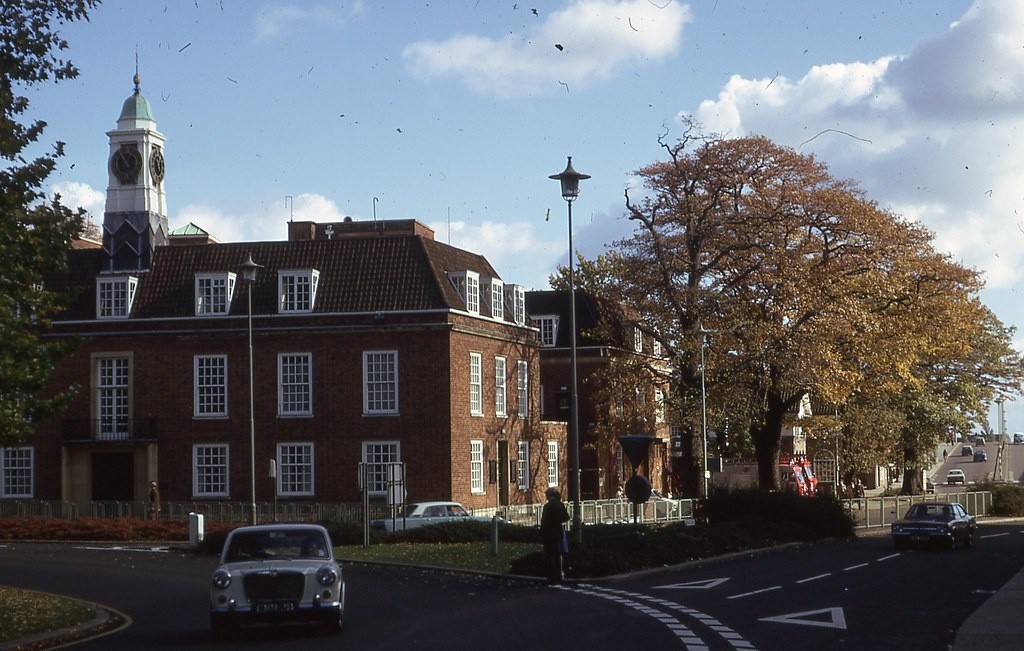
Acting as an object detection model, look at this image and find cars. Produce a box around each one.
[372,501,505,536]
[962,445,973,456]
[1014,432,1024,445]
[927,477,936,494]
[888,502,976,551]
[973,450,988,462]
[975,437,985,448]
[208,521,346,639]
[946,468,966,486]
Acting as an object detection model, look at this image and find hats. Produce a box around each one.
[544,488,561,499]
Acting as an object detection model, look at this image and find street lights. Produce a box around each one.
[694,319,718,495]
[548,154,591,555]
[237,248,265,525]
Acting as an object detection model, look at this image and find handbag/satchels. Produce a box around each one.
[559,524,570,559]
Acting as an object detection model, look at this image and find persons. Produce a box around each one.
[305,536,324,557]
[538,488,570,590]
[616,486,624,498]
[149,482,161,512]
[837,479,865,513]
[943,449,948,462]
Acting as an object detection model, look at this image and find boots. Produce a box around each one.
[544,562,561,583]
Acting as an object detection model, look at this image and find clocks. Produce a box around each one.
[111,146,142,180]
[148,149,165,184]
